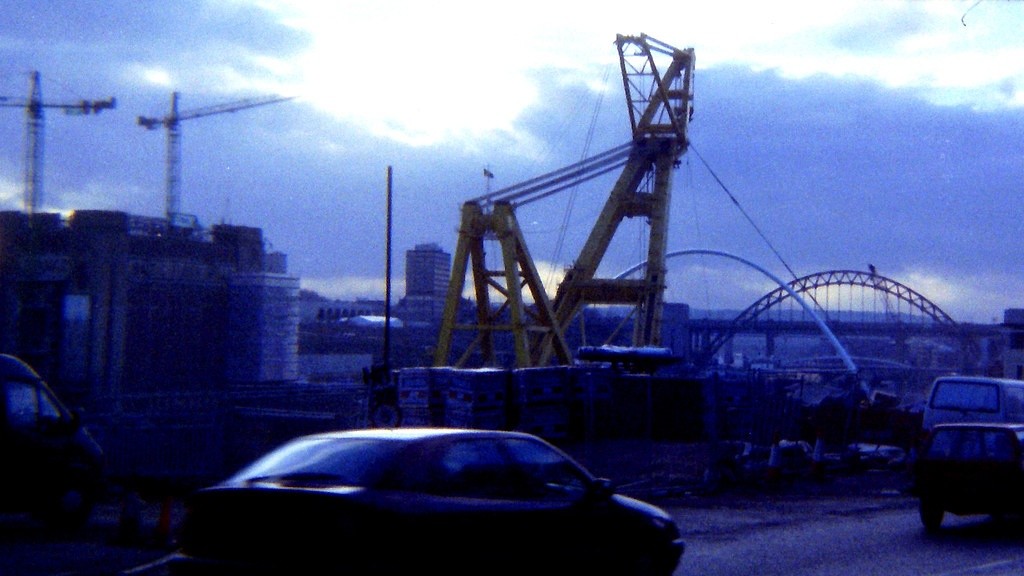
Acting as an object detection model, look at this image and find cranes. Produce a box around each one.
[1,72,114,215]
[138,94,290,225]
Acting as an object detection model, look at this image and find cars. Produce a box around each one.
[190,426,687,576]
[914,422,1023,534]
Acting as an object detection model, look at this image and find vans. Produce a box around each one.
[922,376,1023,431]
[0,352,107,539]
